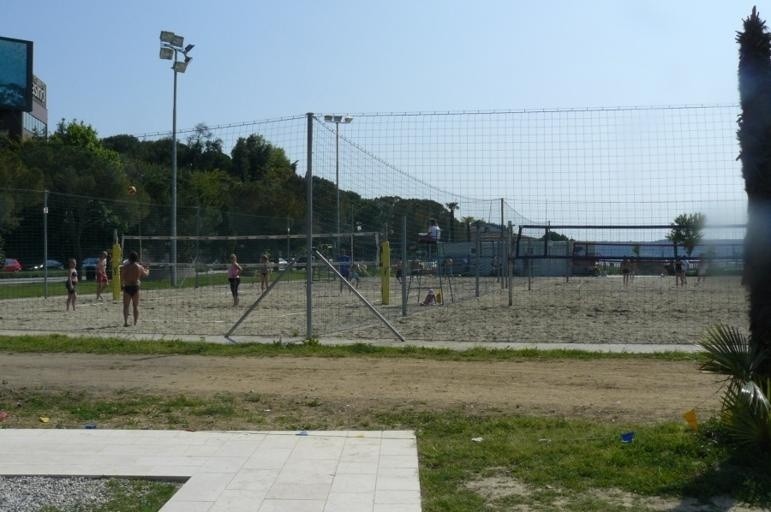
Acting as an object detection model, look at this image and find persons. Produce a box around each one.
[348,264,361,294]
[674,255,683,287]
[416,217,442,244]
[96,250,109,300]
[259,255,272,294]
[228,253,244,307]
[121,251,149,327]
[678,254,690,288]
[65,258,79,311]
[336,247,353,296]
[620,255,633,286]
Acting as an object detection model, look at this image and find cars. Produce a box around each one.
[0,256,129,273]
[263,258,288,269]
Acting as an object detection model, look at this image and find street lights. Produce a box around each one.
[160,29,194,289]
[325,115,353,263]
